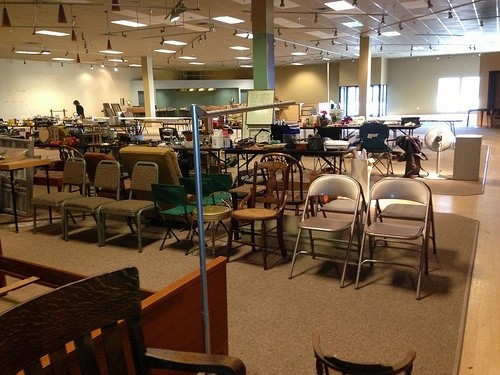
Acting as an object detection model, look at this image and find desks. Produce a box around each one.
[222,142,356,197]
[300,122,421,150]
[467,108,496,128]
[0,158,52,233]
[455,134,484,181]
[144,141,221,178]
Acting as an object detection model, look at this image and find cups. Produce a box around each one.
[212,136,224,149]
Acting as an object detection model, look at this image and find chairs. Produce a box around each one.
[0,117,441,375]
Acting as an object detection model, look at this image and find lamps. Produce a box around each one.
[334,29,338,36]
[169,12,180,22]
[107,39,112,49]
[280,0,285,7]
[279,38,355,63]
[57,0,67,23]
[381,17,386,25]
[313,14,318,23]
[1,3,12,27]
[167,34,207,73]
[71,27,77,41]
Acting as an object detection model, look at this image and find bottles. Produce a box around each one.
[278,119,285,126]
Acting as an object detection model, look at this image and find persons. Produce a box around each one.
[73,100,85,119]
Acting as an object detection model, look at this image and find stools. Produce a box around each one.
[193,206,231,254]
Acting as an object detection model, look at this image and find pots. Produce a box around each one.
[308,138,325,151]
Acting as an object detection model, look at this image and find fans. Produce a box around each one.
[424,127,454,180]
